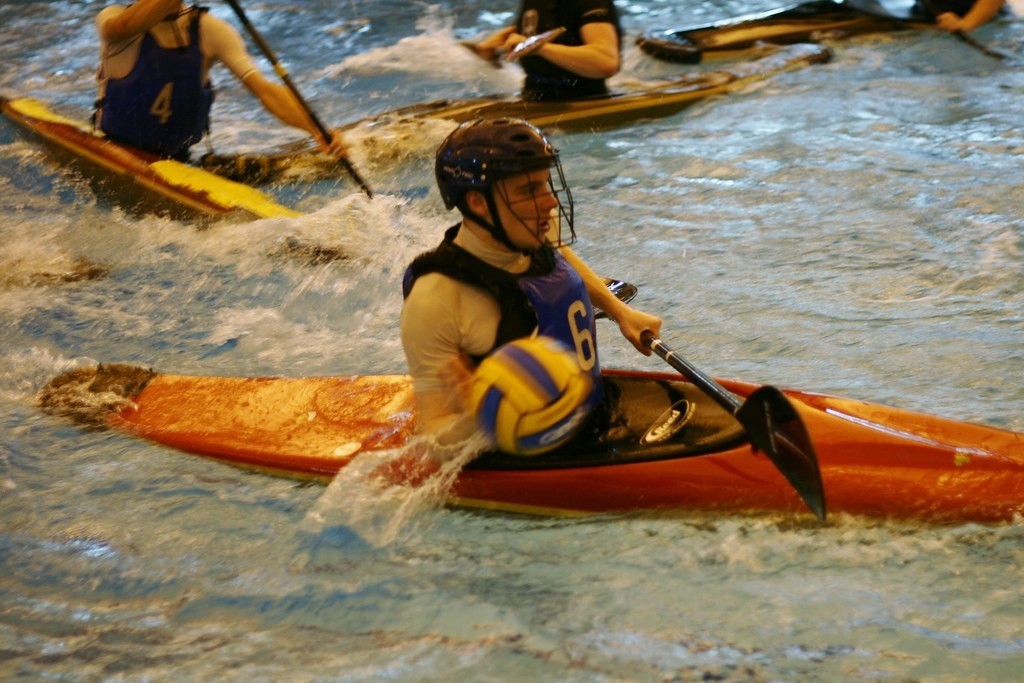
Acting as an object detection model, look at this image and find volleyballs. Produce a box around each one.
[469,336,587,458]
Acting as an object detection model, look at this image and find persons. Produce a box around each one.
[89,0,349,181]
[399,115,662,464]
[909,0,1008,31]
[473,0,624,102]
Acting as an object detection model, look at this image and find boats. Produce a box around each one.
[197,41,832,193]
[633,0,922,68]
[0,86,306,226]
[37,360,1024,525]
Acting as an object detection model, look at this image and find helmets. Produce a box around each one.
[435,116,562,210]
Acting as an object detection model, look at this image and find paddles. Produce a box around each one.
[225,0,376,201]
[915,1,1011,61]
[457,26,568,70]
[589,275,832,522]
